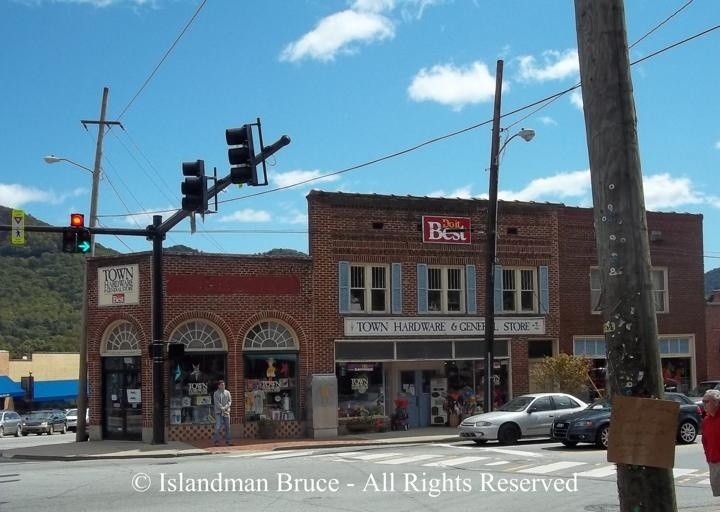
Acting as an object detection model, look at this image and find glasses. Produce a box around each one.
[702,399,709,403]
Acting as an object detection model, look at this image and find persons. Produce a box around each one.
[213,380,234,446]
[702,389,720,497]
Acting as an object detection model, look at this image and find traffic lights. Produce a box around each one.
[181,158,209,214]
[225,123,259,185]
[62,213,92,254]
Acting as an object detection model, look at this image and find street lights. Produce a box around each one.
[42,154,101,443]
[482,128,538,418]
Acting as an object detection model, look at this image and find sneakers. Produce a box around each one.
[215,441,232,446]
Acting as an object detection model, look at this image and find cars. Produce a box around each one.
[0,405,92,437]
[458,392,604,447]
[550,392,707,450]
